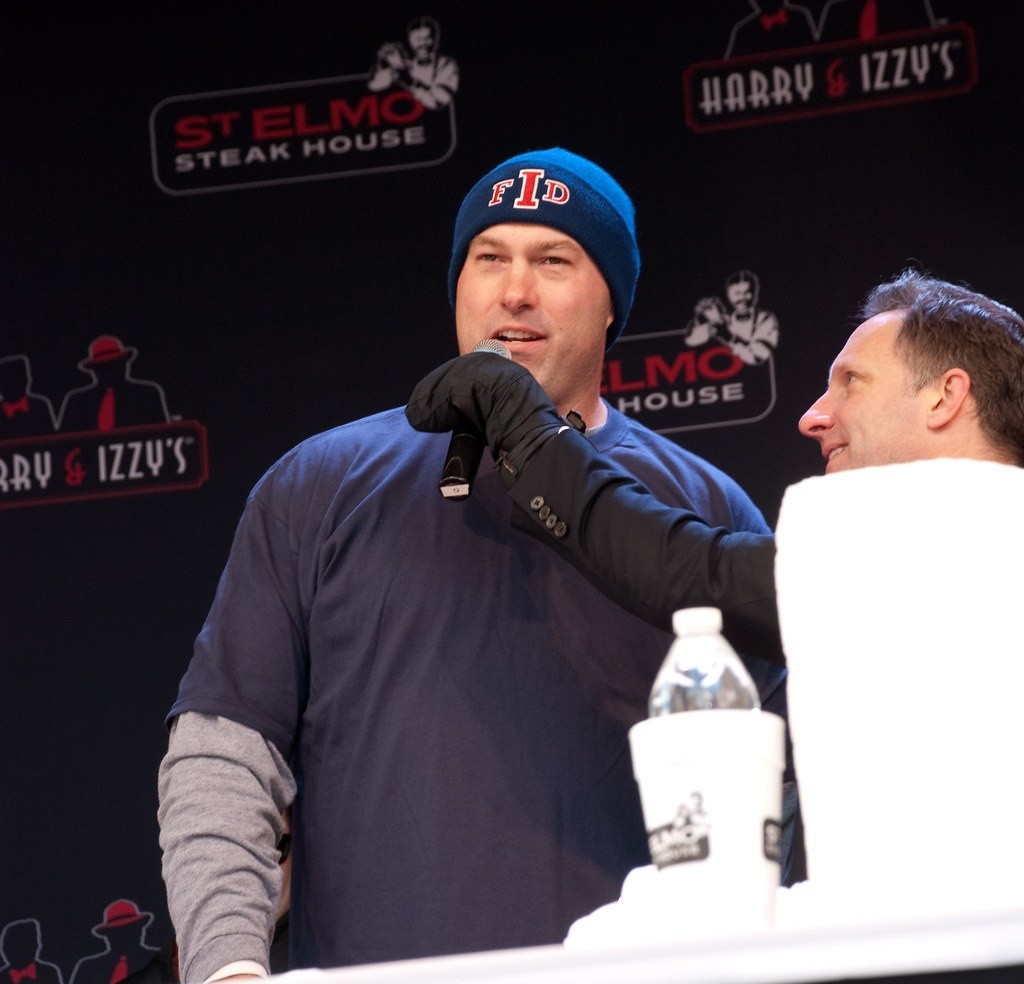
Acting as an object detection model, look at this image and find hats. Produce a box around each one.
[446,147,640,353]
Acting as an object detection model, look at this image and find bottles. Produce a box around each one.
[649,606,760,717]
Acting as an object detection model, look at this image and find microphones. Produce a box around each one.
[439,339,512,503]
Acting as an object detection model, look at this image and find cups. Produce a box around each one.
[627,710,787,892]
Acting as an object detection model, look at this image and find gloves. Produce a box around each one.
[406,351,567,486]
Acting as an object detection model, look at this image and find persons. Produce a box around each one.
[406,265,1023,667]
[157,144,802,984]
[116,807,292,984]
